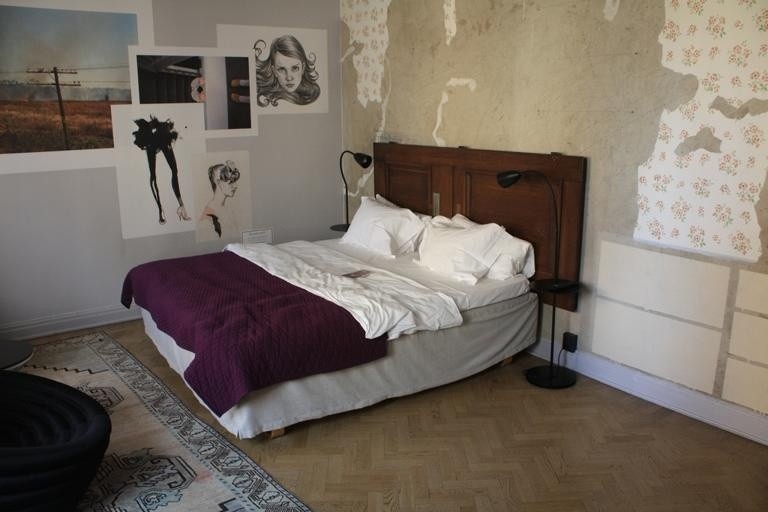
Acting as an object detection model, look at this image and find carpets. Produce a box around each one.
[17,327,312,511]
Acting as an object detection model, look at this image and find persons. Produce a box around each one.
[253,33,321,107]
[199,160,241,219]
[133,115,193,225]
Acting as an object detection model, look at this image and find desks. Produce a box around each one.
[0,338,34,372]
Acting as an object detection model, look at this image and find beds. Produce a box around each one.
[120,141,588,441]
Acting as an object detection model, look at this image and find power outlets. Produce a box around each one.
[562,332,578,353]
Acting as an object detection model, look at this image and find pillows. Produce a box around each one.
[454,213,533,282]
[416,217,501,284]
[342,197,425,259]
[376,194,429,254]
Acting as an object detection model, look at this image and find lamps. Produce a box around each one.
[340,151,372,226]
[497,170,582,390]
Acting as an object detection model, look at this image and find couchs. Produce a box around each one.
[1,372,112,511]
